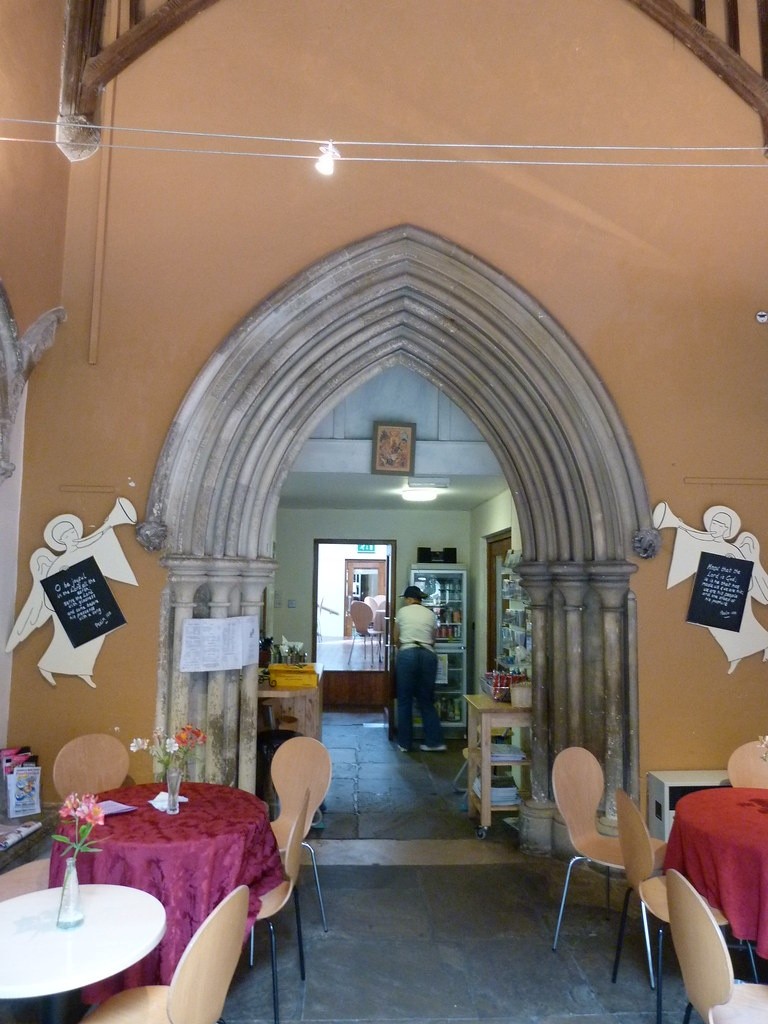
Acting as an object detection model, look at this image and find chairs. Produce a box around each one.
[609,787,759,1024]
[246,736,333,965]
[258,784,314,1024]
[76,884,251,1024]
[347,594,386,668]
[727,739,768,789]
[53,734,129,807]
[550,745,670,990]
[665,867,768,1024]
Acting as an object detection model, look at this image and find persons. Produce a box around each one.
[393,586,447,752]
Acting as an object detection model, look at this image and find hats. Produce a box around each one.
[398,586,421,598]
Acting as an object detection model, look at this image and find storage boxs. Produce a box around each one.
[416,547,457,564]
[479,676,510,701]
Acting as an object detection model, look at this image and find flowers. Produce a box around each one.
[48,792,116,858]
[129,720,209,782]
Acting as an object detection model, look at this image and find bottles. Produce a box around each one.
[415,577,462,600]
[441,697,461,722]
[259,631,307,665]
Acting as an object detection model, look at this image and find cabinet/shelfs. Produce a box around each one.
[408,563,468,728]
[495,553,532,668]
[462,692,531,840]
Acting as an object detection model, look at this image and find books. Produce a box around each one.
[0,746,41,818]
[472,742,526,806]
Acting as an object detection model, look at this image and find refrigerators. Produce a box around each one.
[408,563,473,740]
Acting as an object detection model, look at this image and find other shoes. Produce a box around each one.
[420,745,446,751]
[398,745,409,752]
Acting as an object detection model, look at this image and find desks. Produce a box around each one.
[258,684,314,731]
[374,609,386,631]
[662,787,768,983]
[46,779,284,1007]
[0,883,168,1024]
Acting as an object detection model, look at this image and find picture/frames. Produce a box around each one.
[371,419,417,476]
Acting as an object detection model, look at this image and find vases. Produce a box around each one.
[165,769,183,816]
[57,857,86,929]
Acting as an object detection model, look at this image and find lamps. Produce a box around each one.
[314,138,340,177]
[399,477,450,502]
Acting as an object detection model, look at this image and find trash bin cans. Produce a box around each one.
[257,730,305,776]
